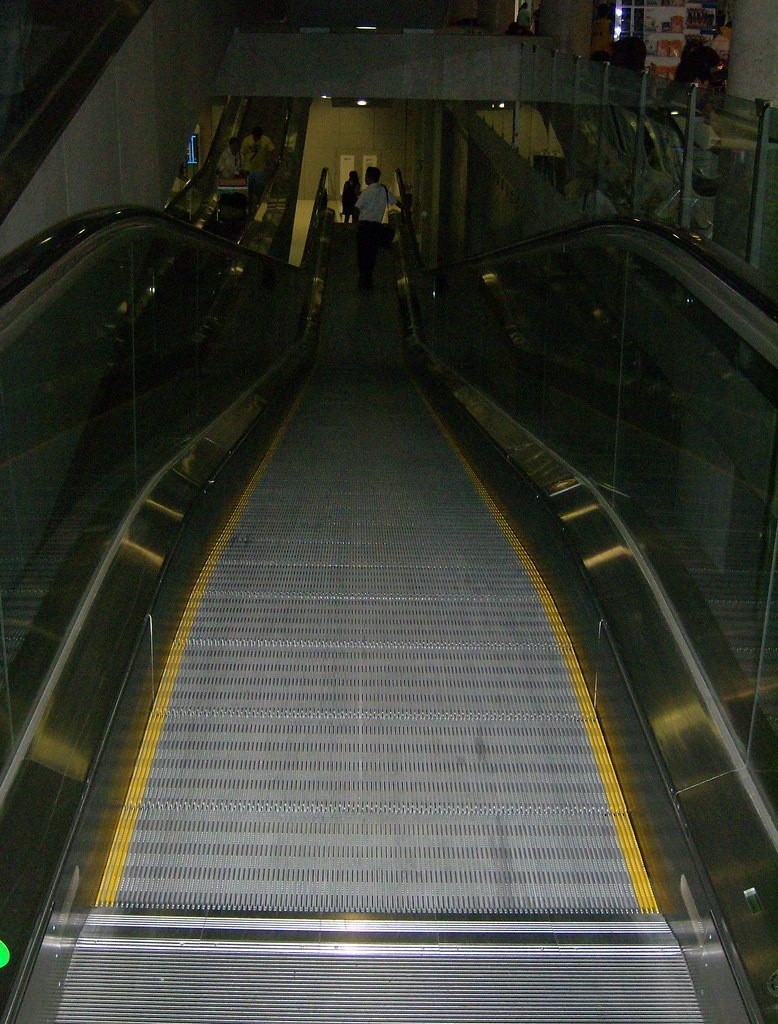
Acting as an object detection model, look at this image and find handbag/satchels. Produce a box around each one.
[379,223,393,250]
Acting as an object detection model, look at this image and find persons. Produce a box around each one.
[341,171,362,224]
[354,166,412,288]
[217,136,250,178]
[239,125,275,175]
[517,2,617,53]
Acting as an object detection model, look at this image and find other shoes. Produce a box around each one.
[357,281,374,293]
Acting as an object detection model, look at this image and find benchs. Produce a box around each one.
[549,75,721,236]
